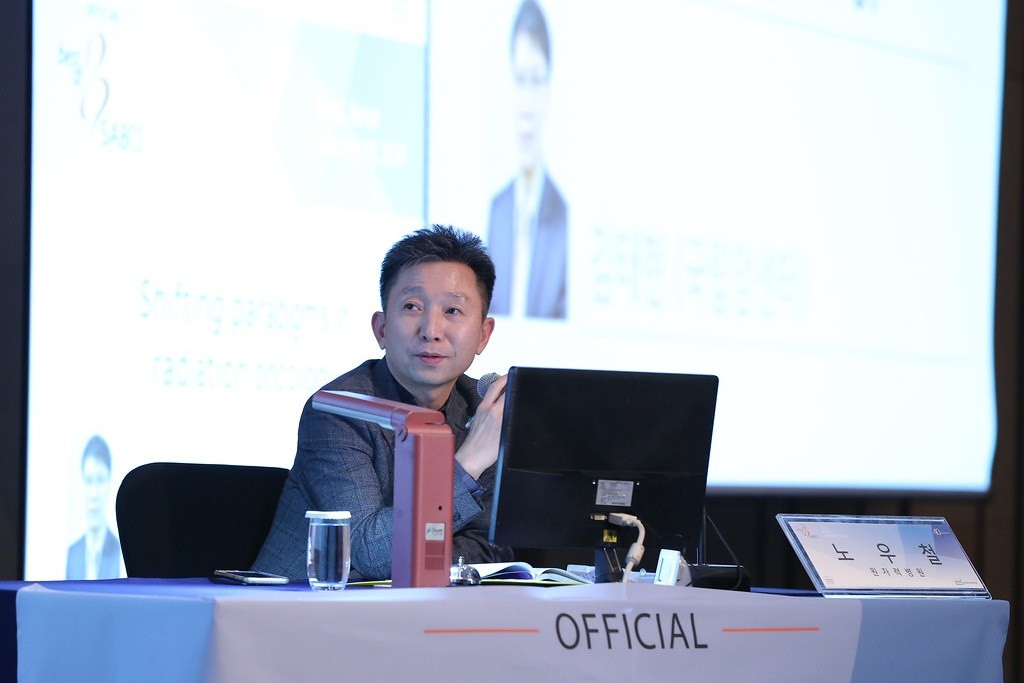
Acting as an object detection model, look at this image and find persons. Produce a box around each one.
[482,0,569,321]
[248,224,508,587]
[64,434,120,581]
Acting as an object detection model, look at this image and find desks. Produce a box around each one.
[0,579,1010,683]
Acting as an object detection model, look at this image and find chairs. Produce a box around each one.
[116,463,291,580]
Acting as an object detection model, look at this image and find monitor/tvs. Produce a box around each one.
[488,367,719,584]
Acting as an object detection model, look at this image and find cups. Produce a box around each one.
[307,510,351,592]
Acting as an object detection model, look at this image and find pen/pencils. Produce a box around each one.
[464,383,508,428]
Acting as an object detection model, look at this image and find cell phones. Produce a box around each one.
[214,568,289,584]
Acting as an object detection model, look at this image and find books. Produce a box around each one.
[450,559,599,594]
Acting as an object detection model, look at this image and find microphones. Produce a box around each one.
[477,371,507,401]
